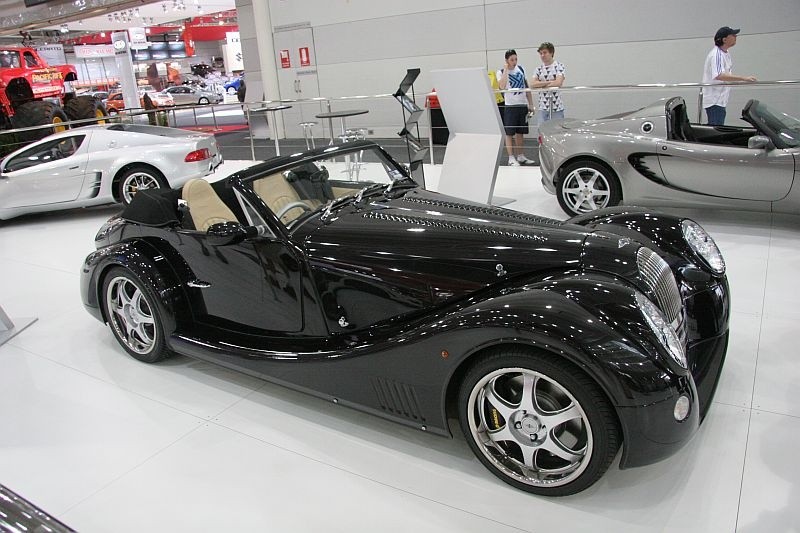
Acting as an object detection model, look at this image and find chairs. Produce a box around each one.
[255,174,304,224]
[182,179,240,232]
[680,112,696,142]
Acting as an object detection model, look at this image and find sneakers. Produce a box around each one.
[508,159,520,166]
[517,156,535,164]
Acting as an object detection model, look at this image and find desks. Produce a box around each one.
[250,105,292,158]
[317,110,368,164]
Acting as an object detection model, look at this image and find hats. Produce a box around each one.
[714,27,740,41]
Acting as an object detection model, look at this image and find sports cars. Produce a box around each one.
[0,122,224,222]
[537,96,799,218]
[80,137,732,498]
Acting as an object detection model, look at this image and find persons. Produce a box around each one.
[496,49,536,166]
[702,27,757,126]
[237,80,248,126]
[529,42,566,124]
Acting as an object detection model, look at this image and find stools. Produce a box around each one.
[299,121,374,182]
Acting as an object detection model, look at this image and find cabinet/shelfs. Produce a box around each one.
[392,68,430,190]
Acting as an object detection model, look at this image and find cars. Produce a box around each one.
[75,55,246,109]
[0,47,79,123]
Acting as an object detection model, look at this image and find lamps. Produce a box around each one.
[107,7,154,26]
[78,20,84,26]
[162,0,202,14]
[60,22,68,33]
